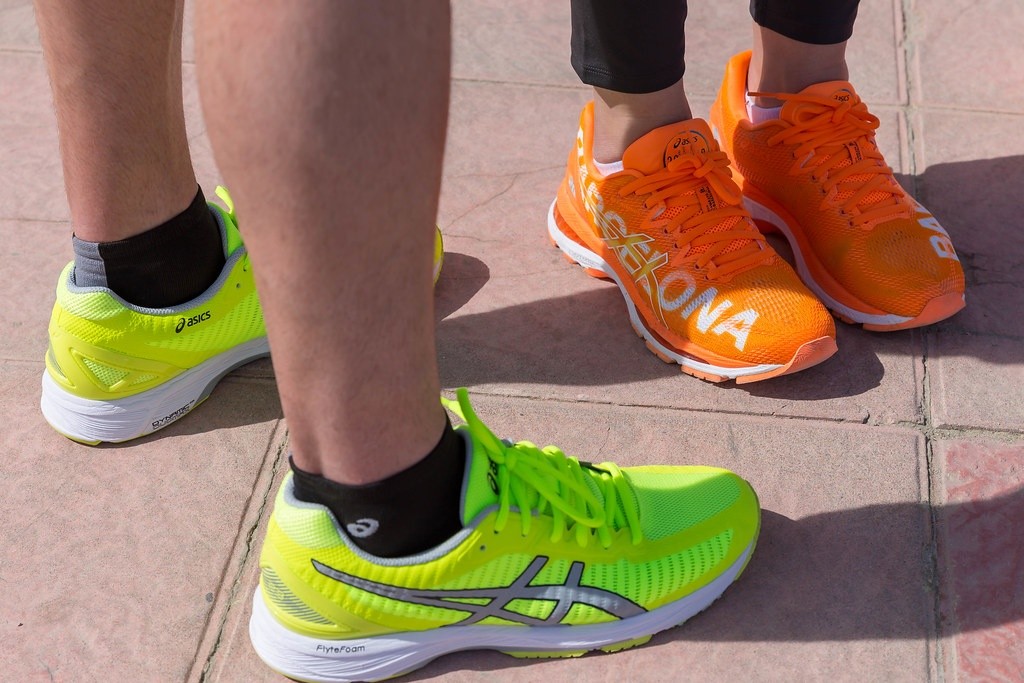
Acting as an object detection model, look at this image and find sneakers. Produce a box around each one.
[545,102,840,385]
[248,389,761,683]
[709,49,967,332]
[41,185,443,448]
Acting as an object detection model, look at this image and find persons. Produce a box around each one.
[41,1,761,681]
[547,0,967,384]
